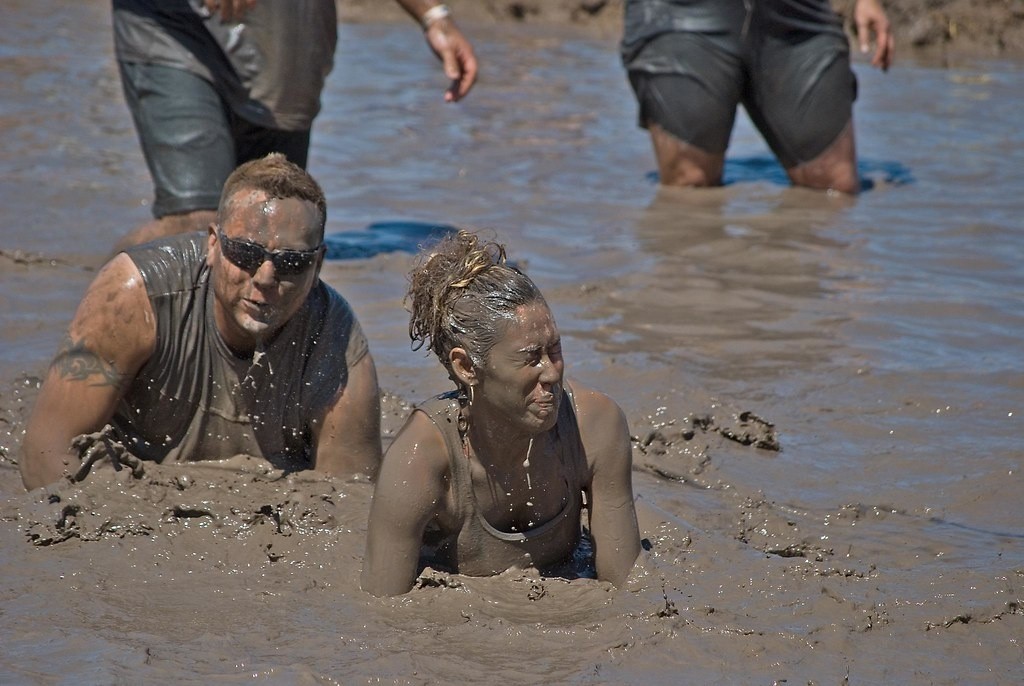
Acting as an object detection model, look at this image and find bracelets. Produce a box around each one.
[421,4,450,29]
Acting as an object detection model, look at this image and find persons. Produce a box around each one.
[111,0,478,255]
[620,0,895,196]
[20,153,382,493]
[359,229,642,597]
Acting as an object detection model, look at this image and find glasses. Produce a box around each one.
[215,223,319,275]
